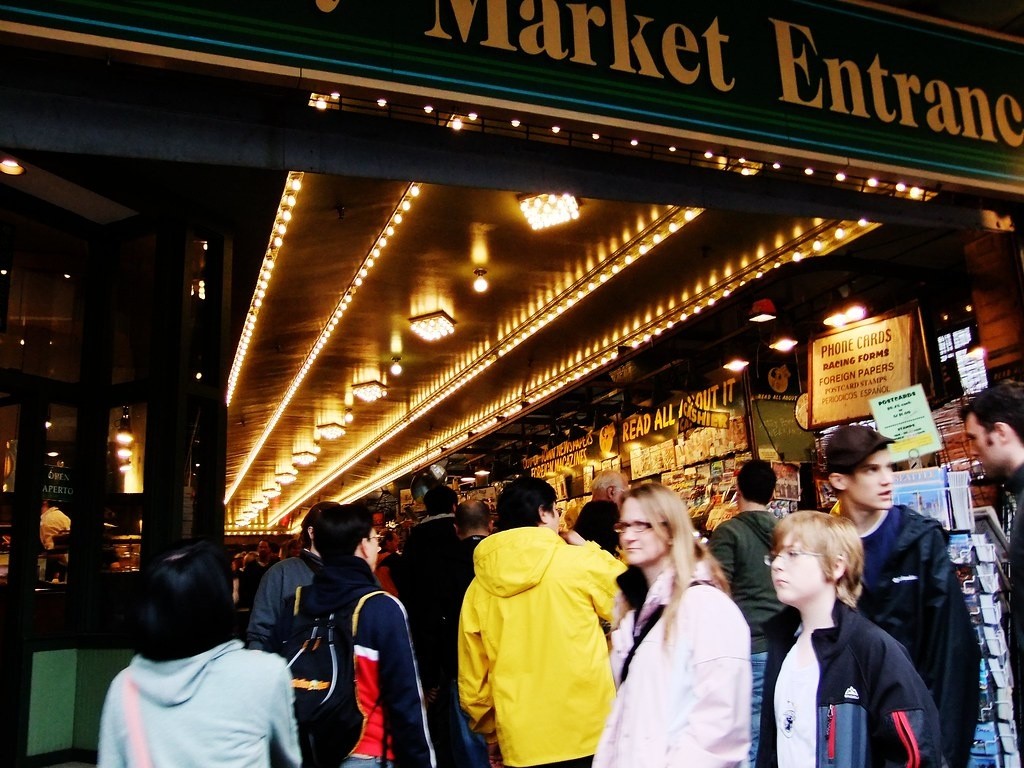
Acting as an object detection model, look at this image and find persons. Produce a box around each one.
[957,380,1024,768]
[227,425,983,768]
[39,494,72,585]
[98,543,303,768]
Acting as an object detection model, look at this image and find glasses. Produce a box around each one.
[544,505,562,517]
[615,521,669,533]
[763,553,825,564]
[364,533,384,542]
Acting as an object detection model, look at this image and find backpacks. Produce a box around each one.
[277,612,365,768]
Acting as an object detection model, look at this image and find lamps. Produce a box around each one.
[442,354,715,492]
[748,298,777,323]
[115,405,133,459]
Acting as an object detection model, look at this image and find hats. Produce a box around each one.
[825,425,896,475]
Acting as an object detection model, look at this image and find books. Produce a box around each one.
[542,417,1022,768]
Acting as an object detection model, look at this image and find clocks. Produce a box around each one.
[794,392,808,431]
